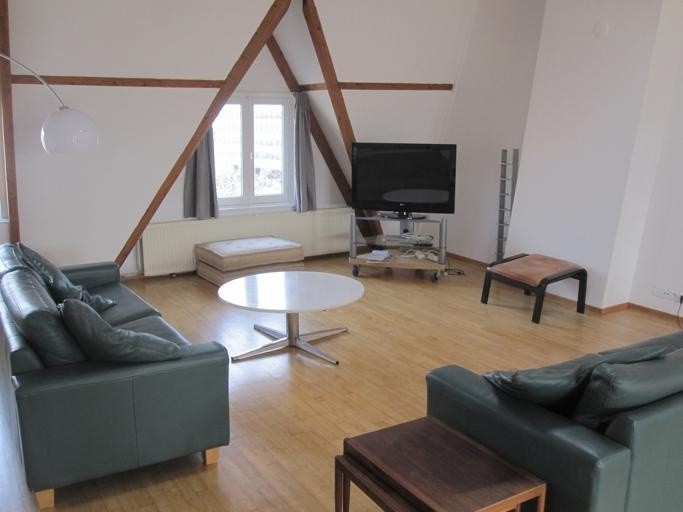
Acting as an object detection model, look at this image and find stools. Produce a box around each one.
[192,234,306,288]
[479,252,587,323]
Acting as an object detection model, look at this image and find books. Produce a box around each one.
[366,250,389,261]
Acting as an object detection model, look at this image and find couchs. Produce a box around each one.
[0,239,231,511]
[426,321,682,512]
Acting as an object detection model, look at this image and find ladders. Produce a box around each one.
[496,148,518,262]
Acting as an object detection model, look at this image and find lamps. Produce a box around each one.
[0,48,100,161]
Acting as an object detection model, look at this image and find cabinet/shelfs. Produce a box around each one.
[347,210,450,284]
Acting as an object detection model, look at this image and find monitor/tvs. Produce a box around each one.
[351,142,457,219]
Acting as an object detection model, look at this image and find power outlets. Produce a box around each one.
[661,287,683,304]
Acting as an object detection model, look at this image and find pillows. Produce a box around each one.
[14,242,117,311]
[59,298,181,367]
[484,343,667,406]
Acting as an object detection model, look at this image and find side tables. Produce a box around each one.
[334,412,549,510]
